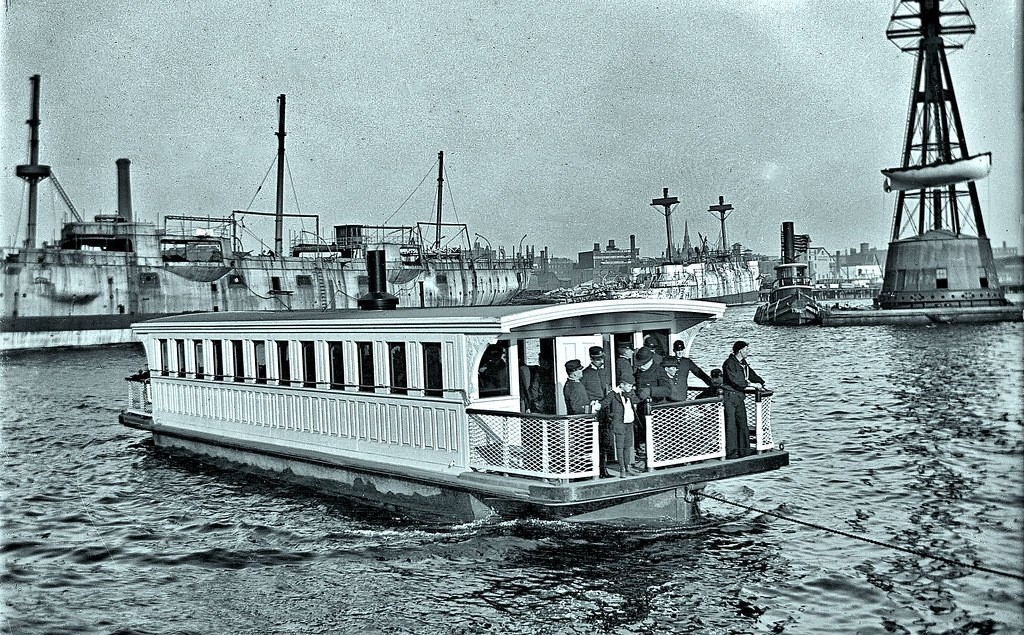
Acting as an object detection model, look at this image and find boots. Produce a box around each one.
[599,453,615,479]
[617,448,640,478]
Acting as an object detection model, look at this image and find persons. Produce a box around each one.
[477,333,774,478]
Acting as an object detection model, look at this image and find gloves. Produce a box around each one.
[590,400,601,410]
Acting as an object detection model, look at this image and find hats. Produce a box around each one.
[538,352,551,361]
[732,341,748,350]
[644,337,659,347]
[619,342,635,350]
[673,340,685,350]
[589,346,606,357]
[710,369,722,378]
[620,373,636,384]
[660,356,681,366]
[635,347,654,366]
[565,359,584,371]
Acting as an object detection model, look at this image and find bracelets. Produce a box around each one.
[763,385,765,386]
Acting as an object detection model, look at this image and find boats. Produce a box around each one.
[117,250,789,530]
[511,271,627,305]
[605,188,766,306]
[754,221,826,325]
[0,74,535,352]
